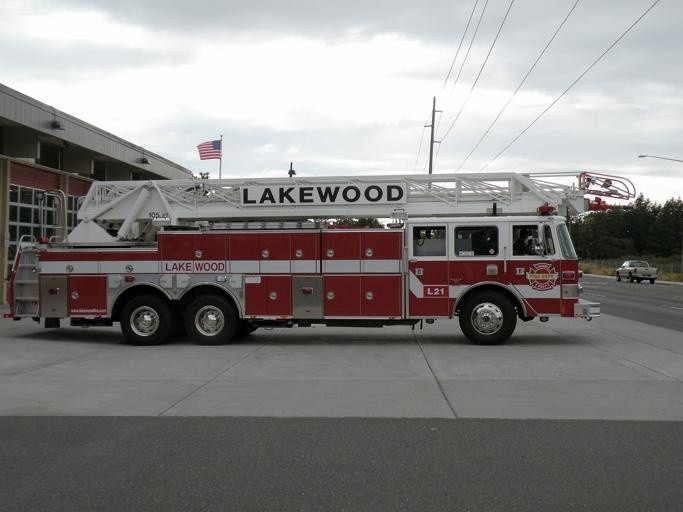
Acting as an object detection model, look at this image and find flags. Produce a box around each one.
[197,139,221,161]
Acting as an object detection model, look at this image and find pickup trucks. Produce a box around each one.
[614,258,660,286]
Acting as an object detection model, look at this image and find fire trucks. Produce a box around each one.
[5,170,642,347]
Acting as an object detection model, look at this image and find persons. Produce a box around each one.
[513,229,537,255]
